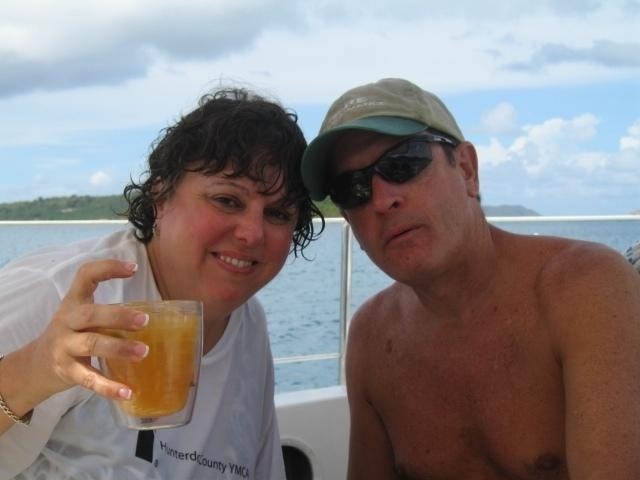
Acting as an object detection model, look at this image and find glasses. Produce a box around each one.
[328,136,457,210]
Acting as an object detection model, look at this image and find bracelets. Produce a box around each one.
[0,354,33,427]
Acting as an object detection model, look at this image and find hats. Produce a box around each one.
[300,79,466,201]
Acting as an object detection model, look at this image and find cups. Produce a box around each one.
[96,299,208,432]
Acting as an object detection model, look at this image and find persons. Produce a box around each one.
[300,77,640,480]
[0,86,325,480]
